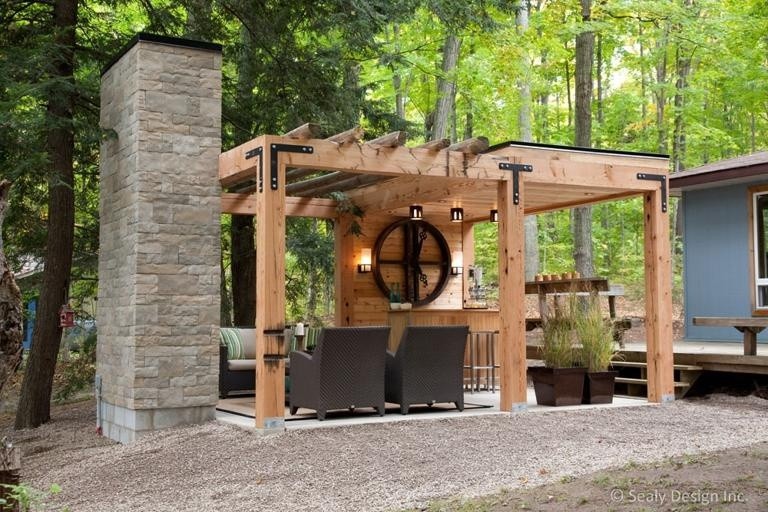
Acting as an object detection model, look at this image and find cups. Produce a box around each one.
[295,323,304,335]
[535,273,581,282]
[390,302,412,310]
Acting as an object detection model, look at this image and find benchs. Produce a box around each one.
[524,277,632,347]
[692,316,768,356]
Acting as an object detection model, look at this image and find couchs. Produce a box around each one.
[219,323,314,399]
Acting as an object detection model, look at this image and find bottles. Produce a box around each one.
[390,282,401,303]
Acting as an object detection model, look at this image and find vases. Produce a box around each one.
[533,272,580,284]
[400,301,412,311]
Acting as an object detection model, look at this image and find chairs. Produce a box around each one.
[289,324,470,422]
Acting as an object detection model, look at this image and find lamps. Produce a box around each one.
[451,249,464,277]
[357,247,372,274]
[408,205,499,223]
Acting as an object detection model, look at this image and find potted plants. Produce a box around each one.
[388,281,402,310]
[526,278,624,407]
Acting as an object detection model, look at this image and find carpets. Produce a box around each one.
[214,399,495,422]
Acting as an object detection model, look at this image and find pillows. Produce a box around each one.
[220,327,246,360]
